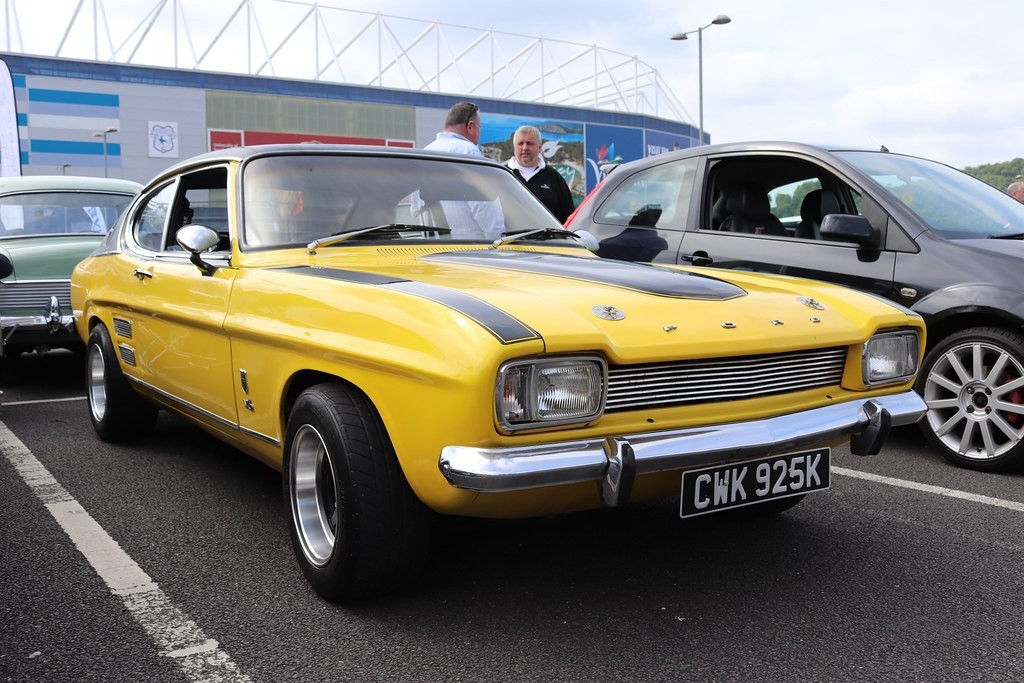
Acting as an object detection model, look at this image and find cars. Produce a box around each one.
[0,175,193,363]
[558,143,1023,474]
[69,144,927,606]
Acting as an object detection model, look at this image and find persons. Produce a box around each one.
[597,204,668,263]
[1007,182,1024,203]
[410,102,506,240]
[504,126,575,225]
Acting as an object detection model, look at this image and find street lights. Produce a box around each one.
[670,14,731,146]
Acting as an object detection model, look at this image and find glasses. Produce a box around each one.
[466,102,478,128]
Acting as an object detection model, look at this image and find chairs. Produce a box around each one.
[719,185,789,238]
[340,195,402,232]
[796,189,842,241]
[23,216,72,231]
